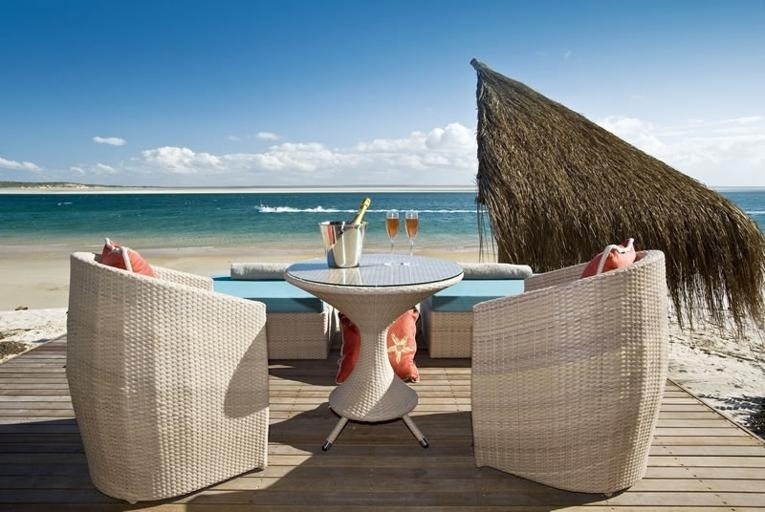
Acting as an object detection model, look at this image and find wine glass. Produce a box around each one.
[383,211,419,267]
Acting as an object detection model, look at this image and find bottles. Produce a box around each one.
[351,197,371,225]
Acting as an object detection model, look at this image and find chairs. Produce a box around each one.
[64,253,271,506]
[470,248,673,500]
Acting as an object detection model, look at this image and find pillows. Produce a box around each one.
[101,236,157,277]
[578,237,638,276]
[334,302,421,385]
[459,262,534,280]
[230,261,288,281]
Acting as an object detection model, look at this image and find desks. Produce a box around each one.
[282,255,466,453]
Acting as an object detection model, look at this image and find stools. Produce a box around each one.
[207,274,334,362]
[420,278,524,358]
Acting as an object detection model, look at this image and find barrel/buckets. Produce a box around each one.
[320,220,367,270]
